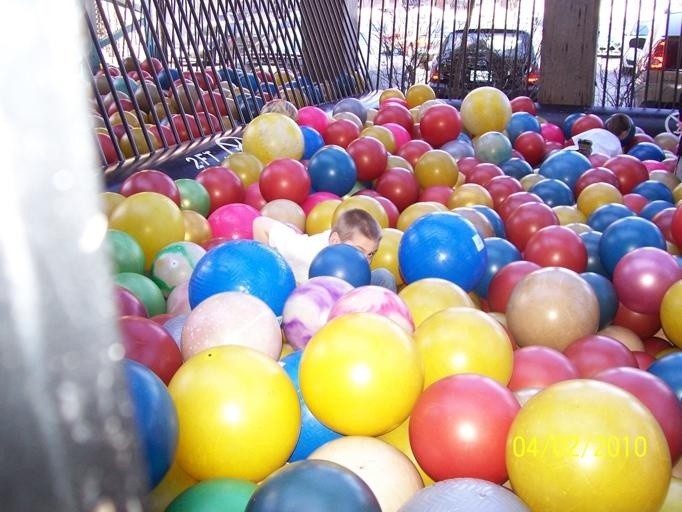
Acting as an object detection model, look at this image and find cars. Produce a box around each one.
[623,37,653,73]
[167,23,241,65]
[241,29,304,66]
[601,46,619,56]
[372,6,459,55]
[633,36,682,107]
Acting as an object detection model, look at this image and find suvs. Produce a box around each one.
[429,30,538,101]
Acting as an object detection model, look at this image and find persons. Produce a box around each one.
[544,112,634,167]
[251,208,383,280]
